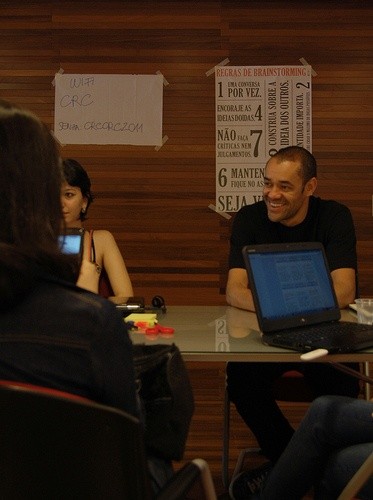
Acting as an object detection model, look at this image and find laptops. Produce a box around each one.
[243,242,373,355]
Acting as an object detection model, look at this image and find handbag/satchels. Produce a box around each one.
[131,343,195,463]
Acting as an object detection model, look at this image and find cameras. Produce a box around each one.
[54,228,83,260]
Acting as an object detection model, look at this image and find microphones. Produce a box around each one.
[152,296,167,312]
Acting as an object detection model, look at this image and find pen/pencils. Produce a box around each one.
[126,320,142,331]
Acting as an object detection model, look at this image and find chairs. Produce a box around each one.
[225,360,373,500]
[0,379,151,500]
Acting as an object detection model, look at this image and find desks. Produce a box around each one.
[122,304,373,494]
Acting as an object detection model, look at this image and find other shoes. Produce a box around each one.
[230,463,273,500]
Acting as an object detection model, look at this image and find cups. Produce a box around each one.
[356,299,373,325]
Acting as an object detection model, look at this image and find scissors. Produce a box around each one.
[145,321,174,335]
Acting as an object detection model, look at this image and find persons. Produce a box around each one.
[260,397,373,500]
[1,101,208,500]
[224,146,359,500]
[57,158,133,305]
